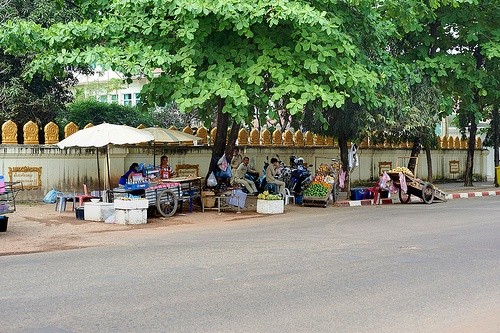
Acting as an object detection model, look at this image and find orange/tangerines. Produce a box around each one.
[311,181,331,188]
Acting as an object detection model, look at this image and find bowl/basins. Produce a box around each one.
[90,199,100,203]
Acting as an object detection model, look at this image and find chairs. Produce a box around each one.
[283,188,295,206]
[52,184,93,212]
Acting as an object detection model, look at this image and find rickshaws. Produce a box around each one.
[384,156,447,204]
[107,176,204,217]
[300,155,341,207]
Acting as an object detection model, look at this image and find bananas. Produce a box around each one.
[257,190,282,199]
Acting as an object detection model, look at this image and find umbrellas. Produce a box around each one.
[139,127,204,171]
[57,121,154,202]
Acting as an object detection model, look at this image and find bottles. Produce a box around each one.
[126,178,147,184]
[163,166,168,171]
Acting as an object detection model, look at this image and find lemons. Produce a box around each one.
[304,184,328,198]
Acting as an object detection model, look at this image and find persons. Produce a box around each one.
[118,163,139,188]
[230,146,260,196]
[155,155,173,179]
[264,154,304,196]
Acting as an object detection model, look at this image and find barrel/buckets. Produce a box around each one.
[0,215,9,231]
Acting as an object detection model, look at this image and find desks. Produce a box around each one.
[161,176,202,212]
[201,196,240,213]
[109,183,180,217]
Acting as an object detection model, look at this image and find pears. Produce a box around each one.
[390,167,413,175]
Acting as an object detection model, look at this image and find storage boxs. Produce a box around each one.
[256,198,284,214]
[351,188,370,200]
[225,190,248,208]
[84,197,148,224]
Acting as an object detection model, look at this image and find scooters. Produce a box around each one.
[241,154,313,195]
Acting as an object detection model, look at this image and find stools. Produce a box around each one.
[266,183,277,194]
